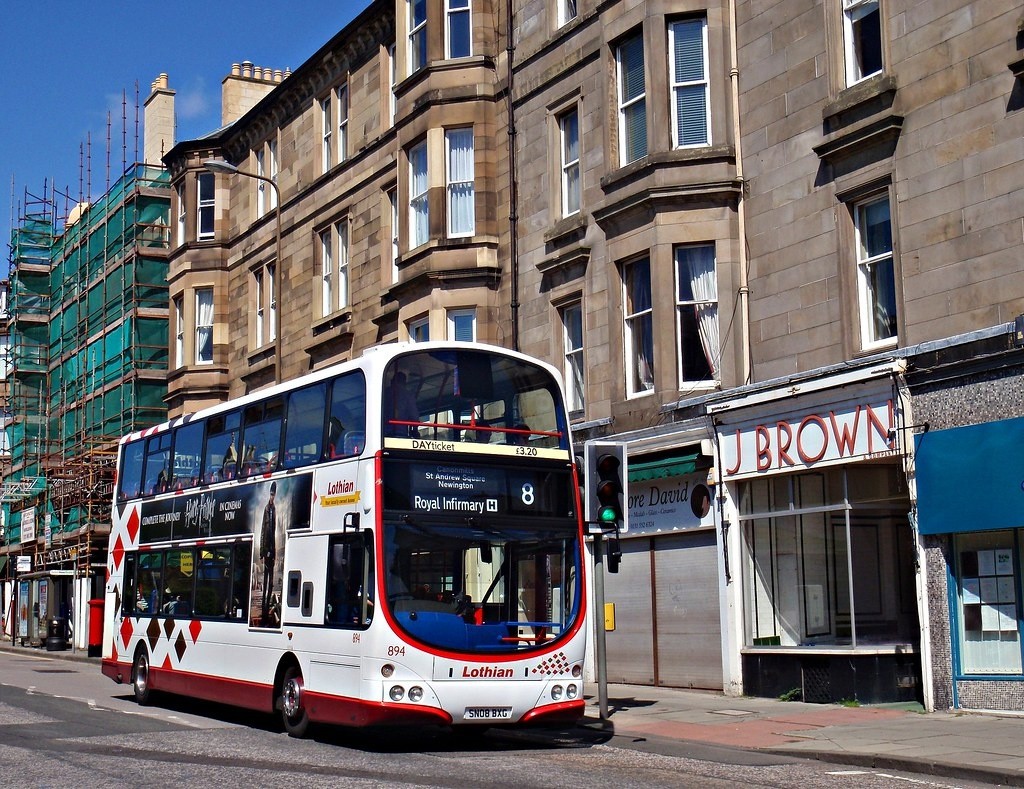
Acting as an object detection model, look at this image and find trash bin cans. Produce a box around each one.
[46,616,67,652]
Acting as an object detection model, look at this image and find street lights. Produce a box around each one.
[201,161,285,385]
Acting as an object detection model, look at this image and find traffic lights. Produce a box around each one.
[572,454,589,536]
[582,436,629,536]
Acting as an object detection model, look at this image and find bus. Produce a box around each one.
[98,340,625,741]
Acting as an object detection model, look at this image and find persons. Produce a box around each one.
[384,371,420,438]
[476,422,492,443]
[335,558,351,606]
[357,543,442,609]
[513,424,531,445]
[163,575,178,592]
[260,482,276,606]
